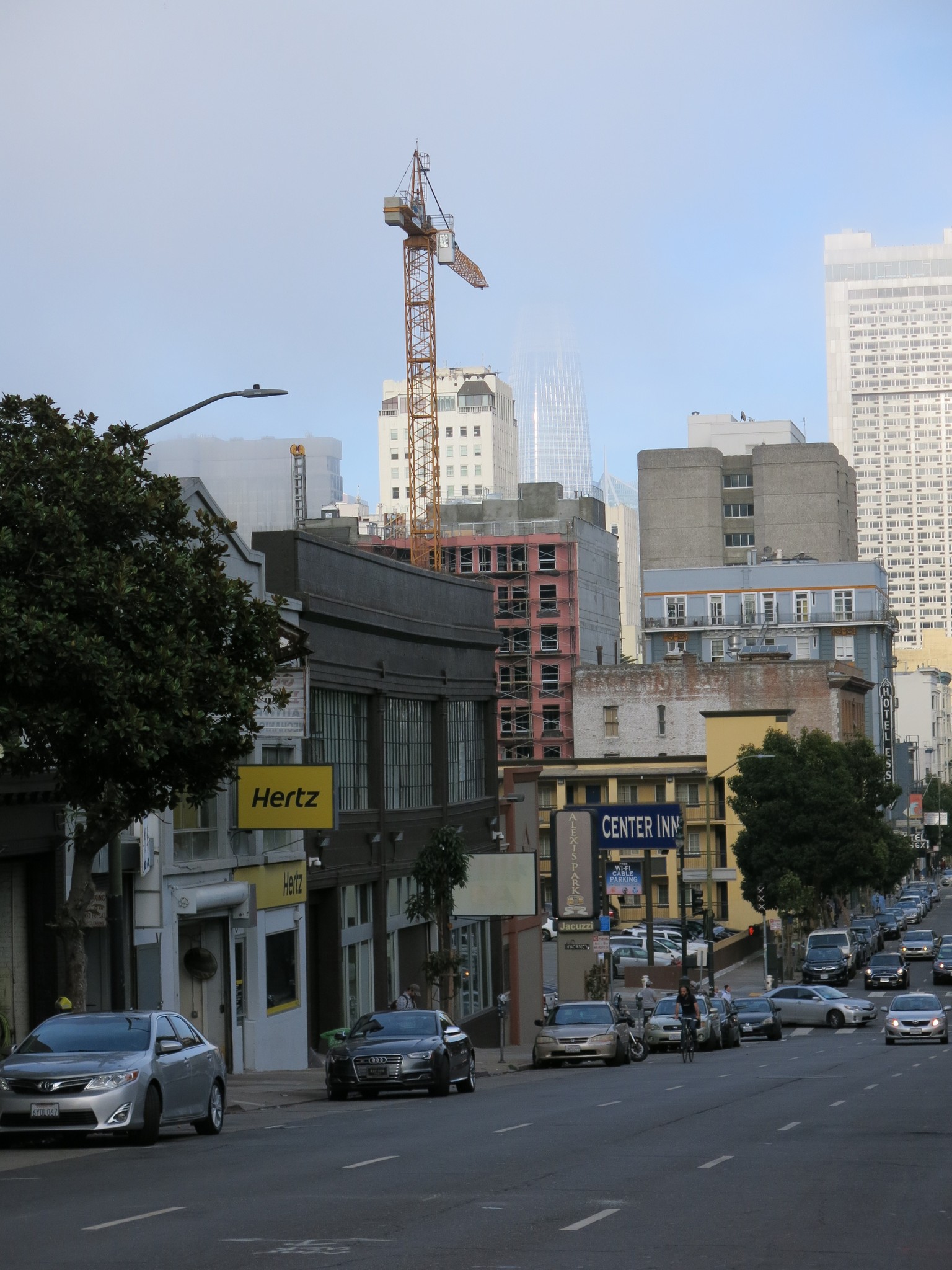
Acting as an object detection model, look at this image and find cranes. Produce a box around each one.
[382,139,489,572]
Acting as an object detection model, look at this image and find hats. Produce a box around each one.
[409,984,421,997]
[646,981,652,985]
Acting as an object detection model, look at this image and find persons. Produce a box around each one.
[395,984,421,1028]
[826,901,833,922]
[714,985,719,997]
[642,981,657,1027]
[673,985,700,1049]
[721,985,731,1004]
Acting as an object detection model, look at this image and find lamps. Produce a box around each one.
[229,829,253,875]
[262,833,324,854]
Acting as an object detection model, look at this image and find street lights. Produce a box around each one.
[705,754,777,997]
[97,384,286,1014]
[906,775,936,882]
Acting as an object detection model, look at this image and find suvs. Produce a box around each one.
[598,917,744,979]
[802,945,852,987]
[642,990,723,1052]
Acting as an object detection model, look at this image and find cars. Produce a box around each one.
[848,880,941,968]
[942,869,952,886]
[324,1008,477,1100]
[531,1000,631,1067]
[730,996,783,1040]
[600,900,621,929]
[863,951,911,990]
[755,983,879,1030]
[542,903,558,941]
[708,996,742,1050]
[932,933,952,985]
[882,993,952,1044]
[0,1009,226,1148]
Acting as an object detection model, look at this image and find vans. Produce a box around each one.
[805,927,861,970]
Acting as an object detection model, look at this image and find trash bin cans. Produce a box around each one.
[320,1027,351,1047]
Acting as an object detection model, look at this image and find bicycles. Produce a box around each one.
[672,1017,699,1063]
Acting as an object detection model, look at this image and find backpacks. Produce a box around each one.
[390,994,415,1011]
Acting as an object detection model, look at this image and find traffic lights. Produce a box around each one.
[691,888,704,915]
[748,925,762,938]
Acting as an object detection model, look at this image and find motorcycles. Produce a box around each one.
[614,993,648,1062]
